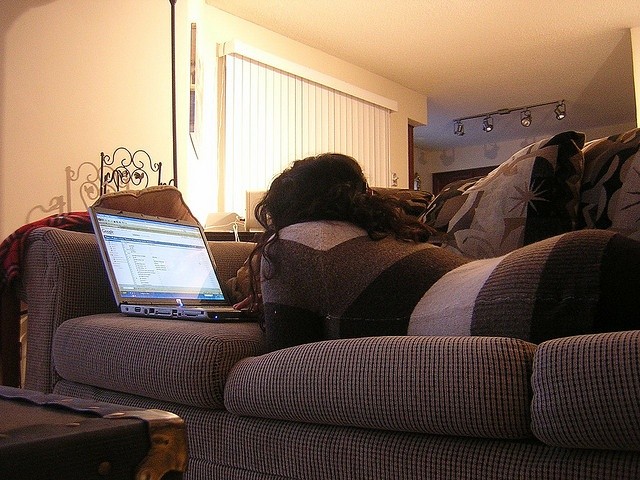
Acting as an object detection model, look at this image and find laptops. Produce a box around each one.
[88,207,254,322]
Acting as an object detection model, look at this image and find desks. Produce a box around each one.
[0,385,189,480]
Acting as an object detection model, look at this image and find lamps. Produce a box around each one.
[519,109,533,128]
[482,114,494,133]
[553,103,567,121]
[453,120,465,136]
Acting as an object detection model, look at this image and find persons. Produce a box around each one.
[232,152,639,353]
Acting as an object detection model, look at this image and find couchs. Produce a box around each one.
[20,220,640,479]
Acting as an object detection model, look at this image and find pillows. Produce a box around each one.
[415,128,586,259]
[365,185,433,232]
[580,127,640,238]
[90,187,202,228]
